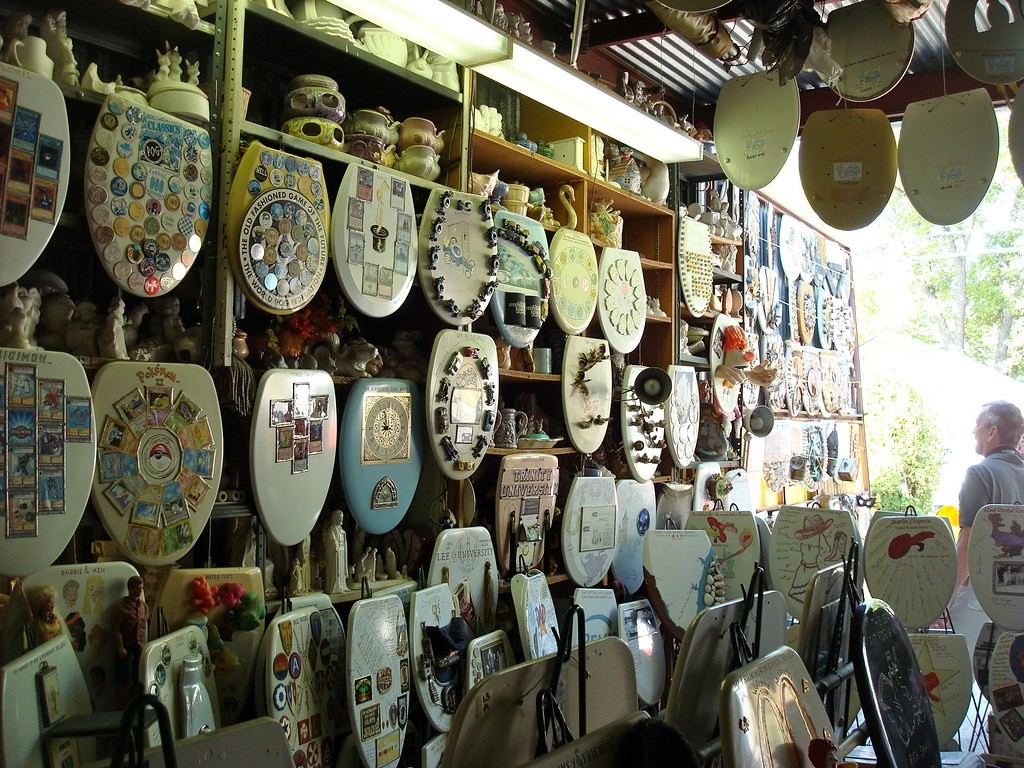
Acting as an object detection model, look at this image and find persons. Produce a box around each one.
[808,738,837,768]
[929,400,1024,628]
[0,9,713,710]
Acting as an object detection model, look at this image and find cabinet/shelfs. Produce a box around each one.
[0,0,868,768]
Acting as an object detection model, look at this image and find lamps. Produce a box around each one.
[329,0,704,164]
